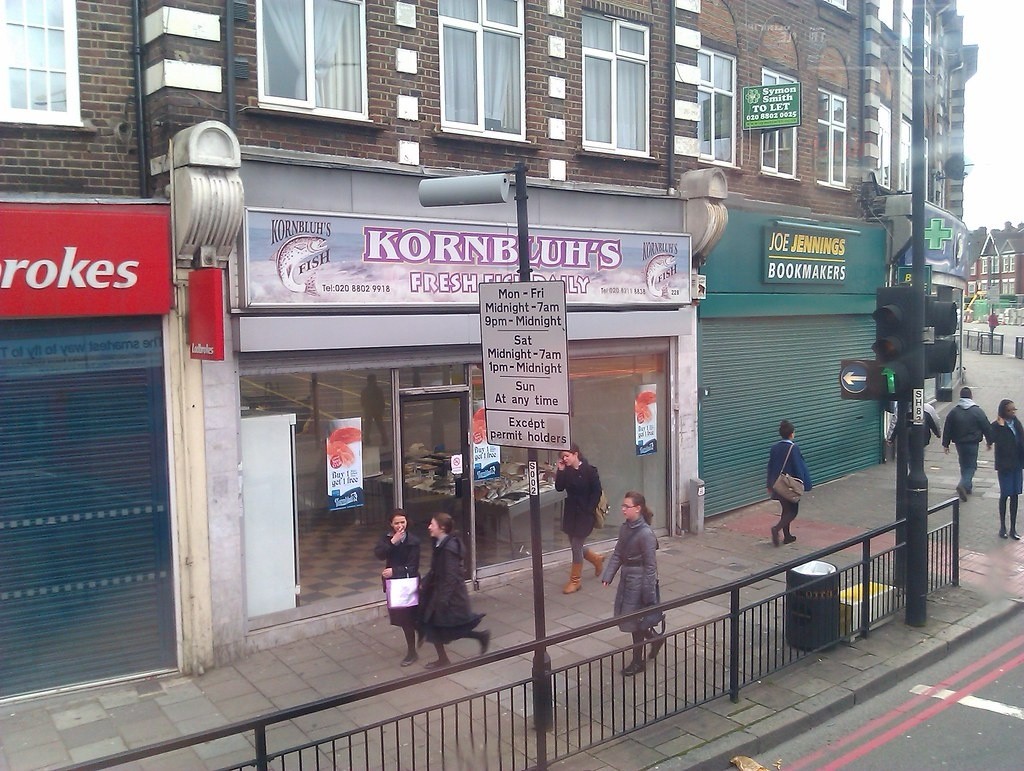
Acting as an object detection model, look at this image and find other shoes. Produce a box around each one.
[784,536,796,544]
[400,652,419,666]
[425,660,452,669]
[999,530,1008,538]
[622,660,647,676]
[772,526,780,545]
[479,630,490,653]
[966,490,973,494]
[1010,531,1020,540]
[957,486,968,502]
[649,634,664,658]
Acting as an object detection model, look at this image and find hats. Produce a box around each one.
[960,387,972,398]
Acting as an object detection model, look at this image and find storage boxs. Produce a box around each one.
[840,581,898,642]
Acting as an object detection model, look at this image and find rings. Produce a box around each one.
[399,528,401,530]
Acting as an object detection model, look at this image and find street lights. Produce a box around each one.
[418,174,555,728]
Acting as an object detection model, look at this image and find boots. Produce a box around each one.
[562,562,583,593]
[585,549,605,576]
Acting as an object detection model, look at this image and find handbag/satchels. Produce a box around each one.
[637,605,665,631]
[386,575,420,610]
[773,474,805,504]
[594,487,612,530]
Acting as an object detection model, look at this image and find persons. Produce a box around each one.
[361,373,388,445]
[416,513,489,669]
[943,387,992,501]
[766,420,812,545]
[987,399,1024,540]
[555,440,605,593]
[988,312,998,332]
[887,399,940,449]
[602,490,664,675]
[375,509,424,666]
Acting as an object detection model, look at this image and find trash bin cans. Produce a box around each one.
[1015,336,1024,359]
[786,559,841,651]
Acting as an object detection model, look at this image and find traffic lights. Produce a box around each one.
[841,287,924,401]
[925,297,958,379]
